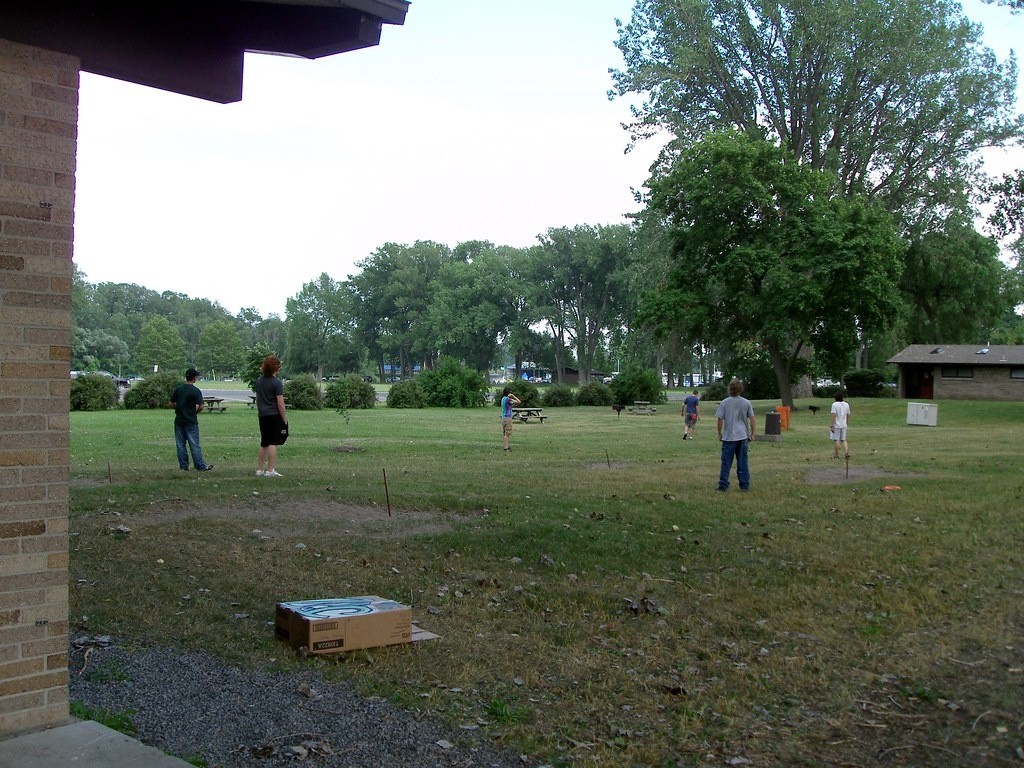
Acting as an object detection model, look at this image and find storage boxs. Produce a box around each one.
[273,596,440,657]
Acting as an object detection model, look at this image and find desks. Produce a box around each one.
[627,401,655,415]
[500,408,545,424]
[196,396,225,414]
[246,395,288,411]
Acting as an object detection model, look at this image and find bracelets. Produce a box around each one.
[830,425,834,427]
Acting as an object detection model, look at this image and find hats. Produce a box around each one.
[186,368,201,379]
[833,391,843,401]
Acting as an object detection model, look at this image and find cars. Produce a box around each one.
[811,378,847,390]
[126,375,144,381]
[70,370,131,391]
[488,375,542,386]
[877,382,898,388]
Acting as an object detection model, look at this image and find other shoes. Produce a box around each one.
[181,467,190,471]
[716,487,728,495]
[740,488,749,492]
[504,447,510,452]
[266,468,284,477]
[202,464,213,471]
[683,432,687,440]
[690,437,693,440]
[831,455,840,459]
[256,470,265,476]
[846,453,850,459]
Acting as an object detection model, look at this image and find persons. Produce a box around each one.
[501,388,521,452]
[830,392,851,459]
[714,379,755,492]
[255,357,290,476]
[169,369,214,471]
[681,390,701,440]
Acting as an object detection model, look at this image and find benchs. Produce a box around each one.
[628,407,633,410]
[196,405,208,407]
[519,415,548,419]
[284,404,292,406]
[650,407,656,411]
[244,402,257,405]
[499,415,528,417]
[207,405,228,409]
